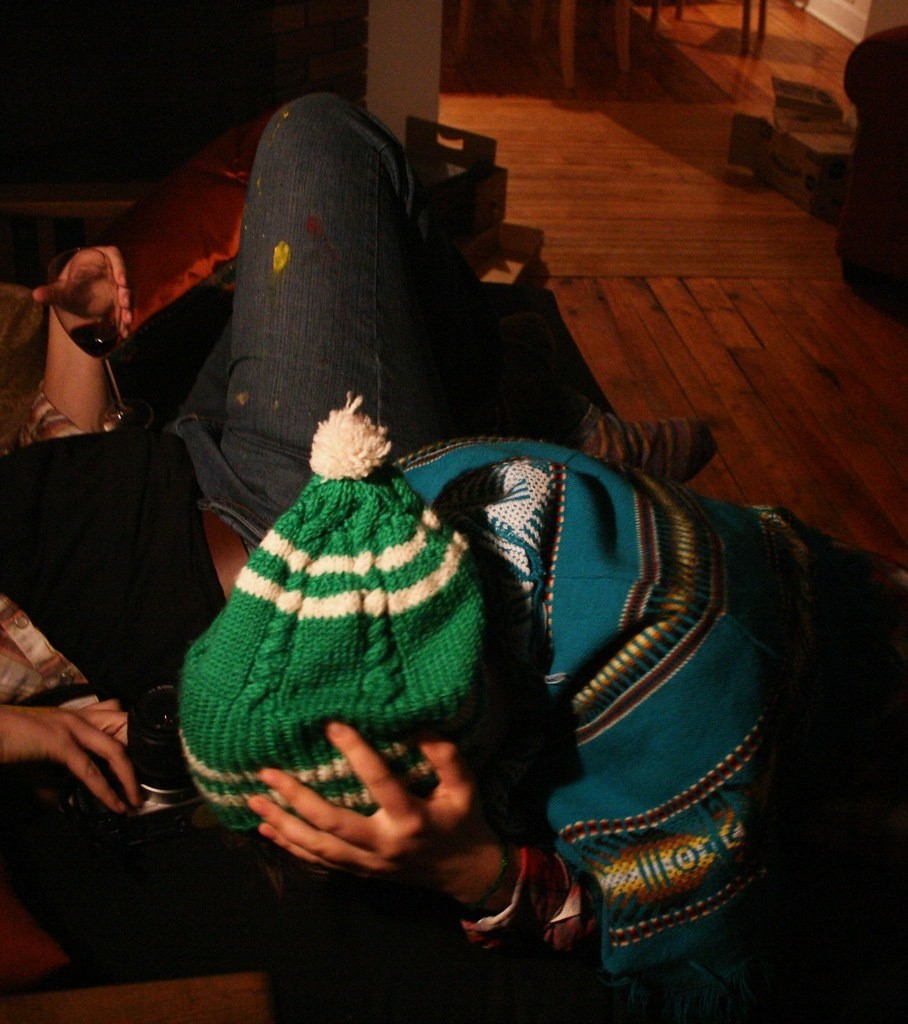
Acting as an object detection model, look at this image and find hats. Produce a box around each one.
[179,400,485,826]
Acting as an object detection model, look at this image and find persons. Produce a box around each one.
[177,395,907,1024]
[1,89,719,818]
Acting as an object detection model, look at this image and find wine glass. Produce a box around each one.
[47,245,155,434]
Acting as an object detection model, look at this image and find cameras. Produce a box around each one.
[74,685,219,849]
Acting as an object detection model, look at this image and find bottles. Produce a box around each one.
[120,682,199,822]
